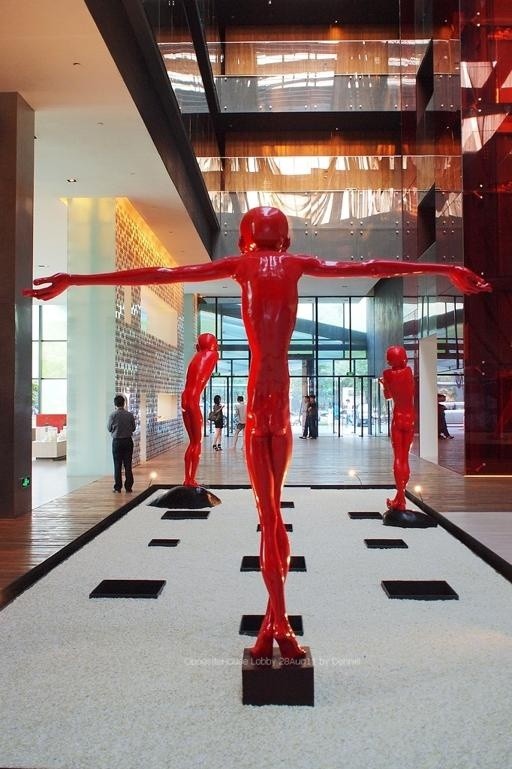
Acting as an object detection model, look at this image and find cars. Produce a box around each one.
[289,403,388,427]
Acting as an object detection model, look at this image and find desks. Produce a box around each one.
[32,440,66,463]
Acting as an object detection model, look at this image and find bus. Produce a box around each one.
[200,384,247,426]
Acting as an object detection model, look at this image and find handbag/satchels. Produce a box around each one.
[208,412,217,421]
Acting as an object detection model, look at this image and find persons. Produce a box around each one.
[346,399,353,426]
[107,396,135,493]
[379,345,416,512]
[438,394,454,439]
[299,395,317,439]
[181,334,218,486]
[20,206,493,659]
[212,395,225,450]
[233,396,246,448]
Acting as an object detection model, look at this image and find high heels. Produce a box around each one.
[212,444,223,451]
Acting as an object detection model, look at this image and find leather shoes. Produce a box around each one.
[126,487,133,493]
[112,489,121,493]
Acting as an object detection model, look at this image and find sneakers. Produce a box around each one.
[299,436,316,440]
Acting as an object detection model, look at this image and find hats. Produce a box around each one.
[304,395,315,398]
